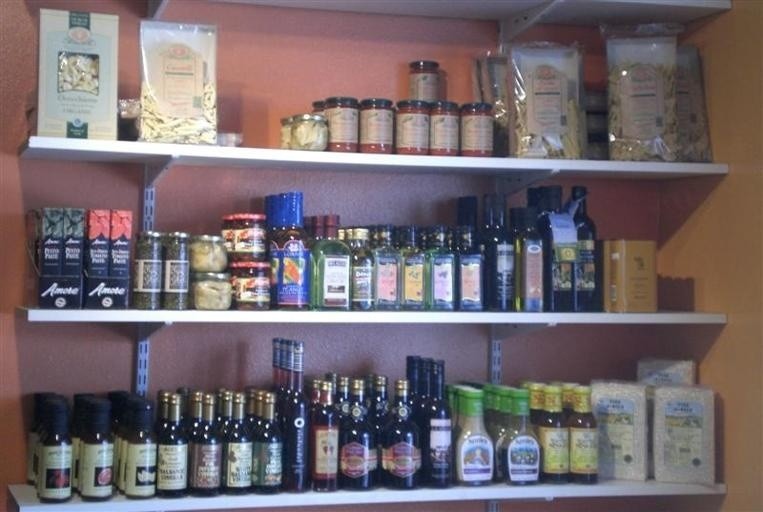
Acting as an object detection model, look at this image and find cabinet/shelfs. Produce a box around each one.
[7,0,732,512]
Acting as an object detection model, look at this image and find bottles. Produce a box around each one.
[454,183,600,311]
[308,370,422,492]
[264,190,311,310]
[443,381,542,486]
[302,212,353,312]
[25,389,157,503]
[157,385,283,500]
[405,354,455,488]
[339,224,484,312]
[521,381,600,486]
[278,97,495,157]
[219,212,270,310]
[272,336,307,493]
[132,230,231,311]
[407,59,440,103]
